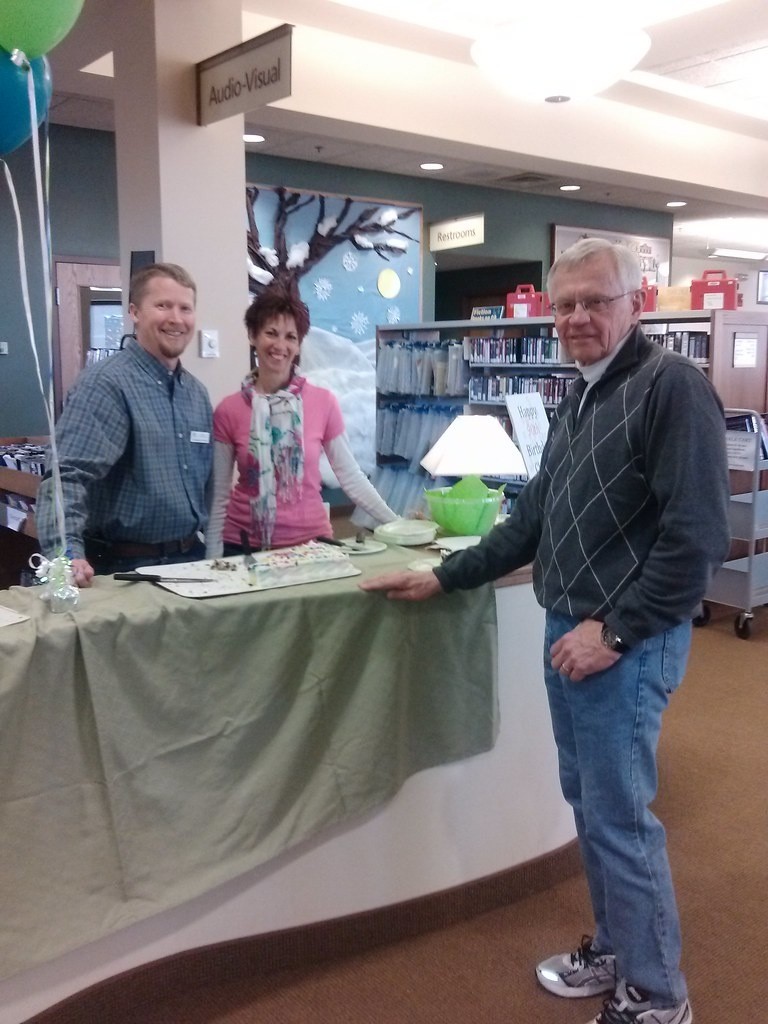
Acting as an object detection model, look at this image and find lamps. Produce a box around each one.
[469,21,653,105]
[419,414,526,536]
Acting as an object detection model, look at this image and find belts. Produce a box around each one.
[109,533,196,559]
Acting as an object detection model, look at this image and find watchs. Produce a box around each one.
[600,623,631,656]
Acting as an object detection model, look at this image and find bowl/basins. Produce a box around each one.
[423,487,506,536]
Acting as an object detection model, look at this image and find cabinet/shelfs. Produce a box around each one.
[374,309,768,641]
[0,436,54,539]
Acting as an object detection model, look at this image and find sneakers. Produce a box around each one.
[582,961,692,1024]
[536,934,625,998]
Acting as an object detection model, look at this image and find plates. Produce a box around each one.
[338,539,387,556]
[371,519,482,572]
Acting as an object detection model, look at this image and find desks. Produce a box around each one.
[1,535,583,1024]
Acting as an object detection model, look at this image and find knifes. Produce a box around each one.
[112,571,216,583]
[317,535,368,551]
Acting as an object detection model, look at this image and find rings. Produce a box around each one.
[562,663,567,672]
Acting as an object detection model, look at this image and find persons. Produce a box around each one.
[356,238,730,1024]
[206,289,402,558]
[36,263,213,590]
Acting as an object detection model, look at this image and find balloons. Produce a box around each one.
[0,0,85,159]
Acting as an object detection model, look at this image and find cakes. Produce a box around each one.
[247,540,349,586]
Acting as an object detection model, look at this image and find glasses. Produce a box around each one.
[548,290,634,314]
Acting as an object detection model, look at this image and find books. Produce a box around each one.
[0,443,46,511]
[645,333,707,359]
[470,335,574,450]
[725,414,768,460]
